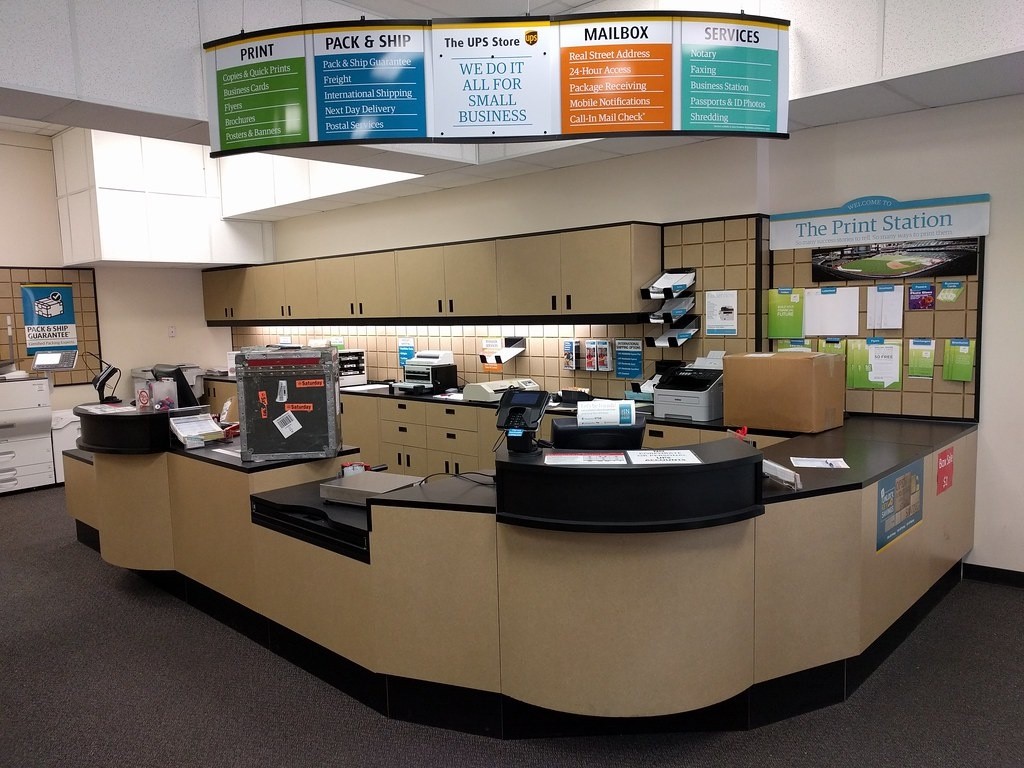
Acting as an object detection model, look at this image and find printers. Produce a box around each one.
[653,356,724,421]
[0,349,79,494]
[392,350,457,394]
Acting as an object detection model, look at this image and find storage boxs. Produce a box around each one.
[639,267,696,300]
[638,291,696,323]
[721,351,845,433]
[479,336,526,364]
[642,314,700,348]
[625,390,653,401]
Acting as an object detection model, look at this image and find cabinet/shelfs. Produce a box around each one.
[200,221,663,325]
[199,381,787,477]
